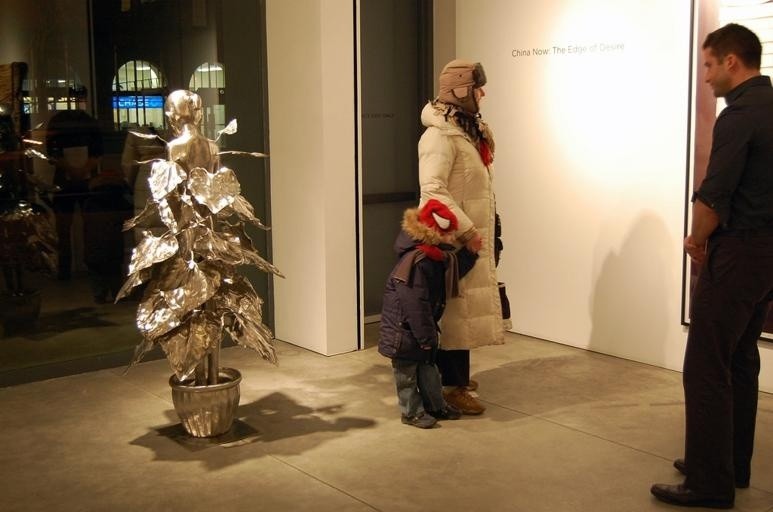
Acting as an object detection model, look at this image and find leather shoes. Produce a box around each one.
[650,459,750,510]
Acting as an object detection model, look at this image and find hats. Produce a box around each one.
[437,59,488,114]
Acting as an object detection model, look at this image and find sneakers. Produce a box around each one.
[427,379,486,421]
[418,199,458,236]
[401,411,437,429]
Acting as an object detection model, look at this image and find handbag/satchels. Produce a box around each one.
[497,280,511,321]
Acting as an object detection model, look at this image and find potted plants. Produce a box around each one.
[0,121,63,338]
[115,117,286,437]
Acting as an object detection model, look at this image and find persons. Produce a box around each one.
[414,57,512,418]
[119,115,179,287]
[376,197,484,429]
[44,85,110,305]
[648,20,772,510]
